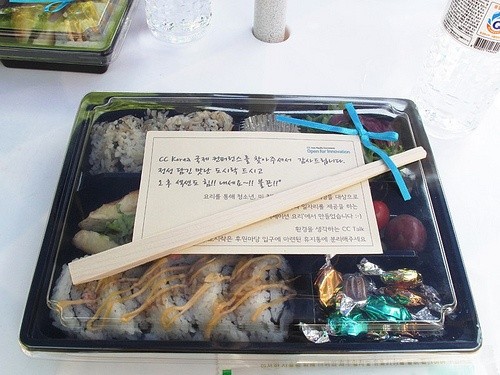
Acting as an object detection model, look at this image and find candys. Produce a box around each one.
[298,254,454,344]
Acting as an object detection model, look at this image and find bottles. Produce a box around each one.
[412,1,500,139]
[145,0,211,43]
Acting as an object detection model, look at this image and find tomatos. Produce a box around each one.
[372,199,390,230]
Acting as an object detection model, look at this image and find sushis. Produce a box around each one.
[88,110,236,174]
[49,254,295,344]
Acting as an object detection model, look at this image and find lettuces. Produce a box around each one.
[309,102,406,180]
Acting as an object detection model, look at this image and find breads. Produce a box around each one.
[72,191,139,253]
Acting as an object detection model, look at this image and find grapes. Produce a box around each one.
[385,213,428,254]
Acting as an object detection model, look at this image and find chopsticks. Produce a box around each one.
[69,145,427,285]
